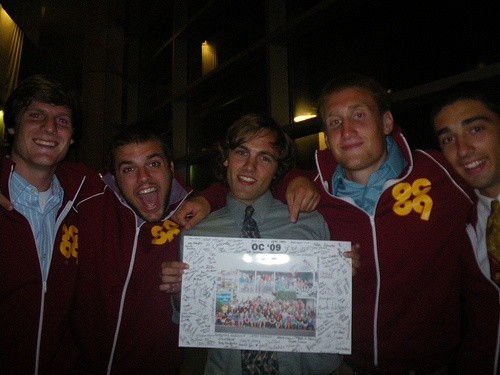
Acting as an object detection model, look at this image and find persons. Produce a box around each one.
[214,270,317,333]
[429,77,500,375]
[159,113,361,375]
[1,118,321,375]
[1,74,101,374]
[172,74,477,375]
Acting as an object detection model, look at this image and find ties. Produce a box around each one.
[486,200,500,287]
[239,206,279,375]
